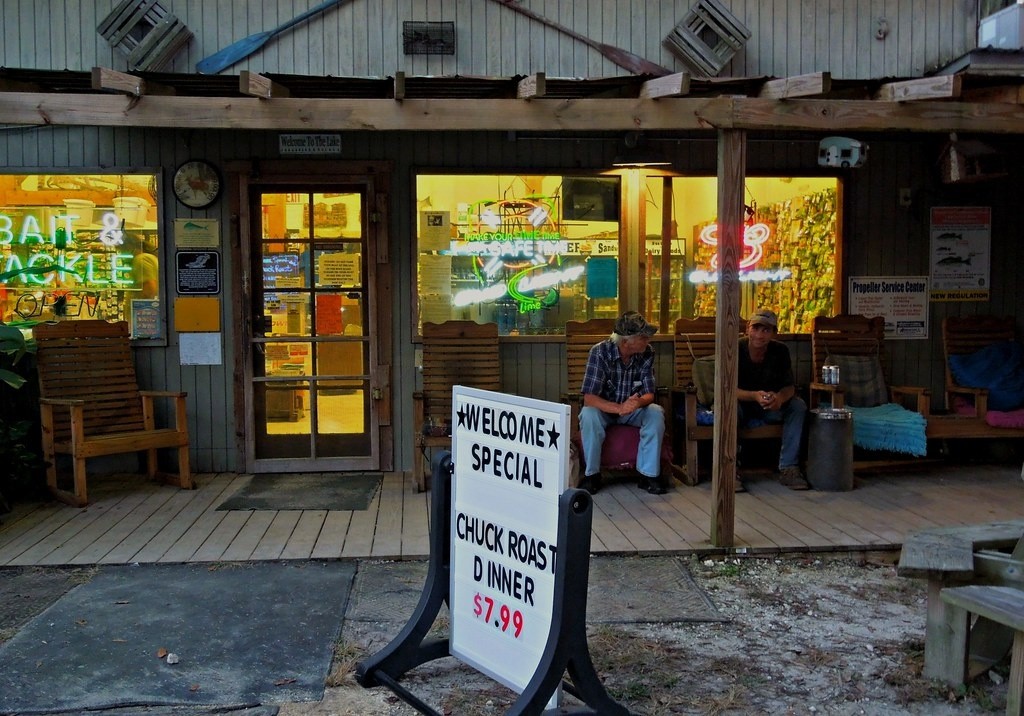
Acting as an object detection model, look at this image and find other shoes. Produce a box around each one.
[637,476,666,494]
[578,473,602,494]
[735,471,745,492]
[780,469,808,489]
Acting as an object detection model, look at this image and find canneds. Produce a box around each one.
[830,366,839,384]
[822,366,830,384]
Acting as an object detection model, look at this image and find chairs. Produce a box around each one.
[809,313,938,476]
[664,316,802,486]
[942,315,1024,437]
[411,320,501,495]
[32,320,198,508]
[558,318,676,491]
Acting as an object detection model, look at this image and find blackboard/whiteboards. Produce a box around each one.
[449,384,574,713]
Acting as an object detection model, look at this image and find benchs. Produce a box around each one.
[940,585,1024,716]
[896,518,1024,684]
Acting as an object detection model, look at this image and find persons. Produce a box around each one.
[576,309,669,495]
[735,309,809,494]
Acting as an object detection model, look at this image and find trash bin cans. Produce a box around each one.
[808,407,855,493]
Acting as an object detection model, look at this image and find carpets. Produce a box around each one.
[215,473,384,512]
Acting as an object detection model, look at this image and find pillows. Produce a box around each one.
[691,354,715,408]
[949,342,1024,412]
[823,354,888,408]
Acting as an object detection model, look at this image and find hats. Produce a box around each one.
[749,310,777,327]
[613,311,658,336]
